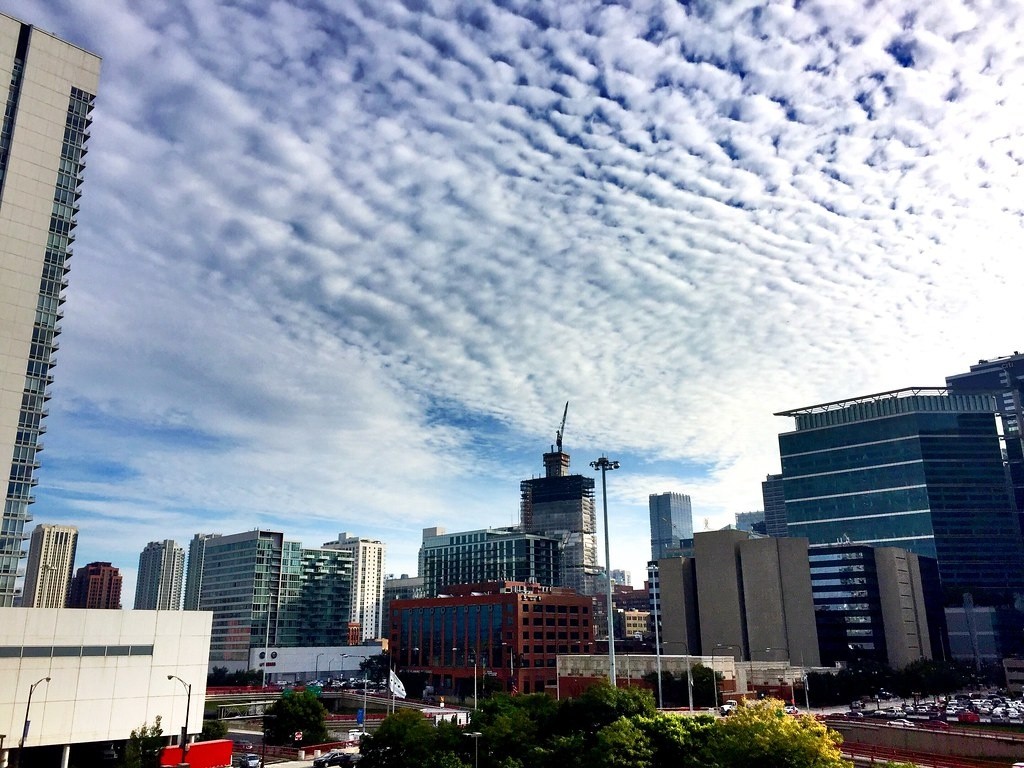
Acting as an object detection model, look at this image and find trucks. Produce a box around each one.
[719,700,739,716]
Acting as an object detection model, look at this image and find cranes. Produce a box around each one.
[555,400,569,452]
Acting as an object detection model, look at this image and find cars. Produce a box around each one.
[886,719,915,727]
[829,692,1024,722]
[785,706,798,714]
[240,754,260,768]
[234,740,253,752]
[340,753,361,768]
[274,680,386,688]
[313,752,345,768]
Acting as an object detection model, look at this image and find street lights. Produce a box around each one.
[502,643,514,688]
[414,647,421,698]
[664,641,694,716]
[452,648,477,711]
[262,593,276,686]
[471,731,483,768]
[589,451,620,689]
[316,653,324,680]
[167,675,192,763]
[342,654,367,734]
[16,677,52,767]
[716,643,744,708]
[712,647,733,713]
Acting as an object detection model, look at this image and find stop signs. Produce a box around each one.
[296,732,302,740]
